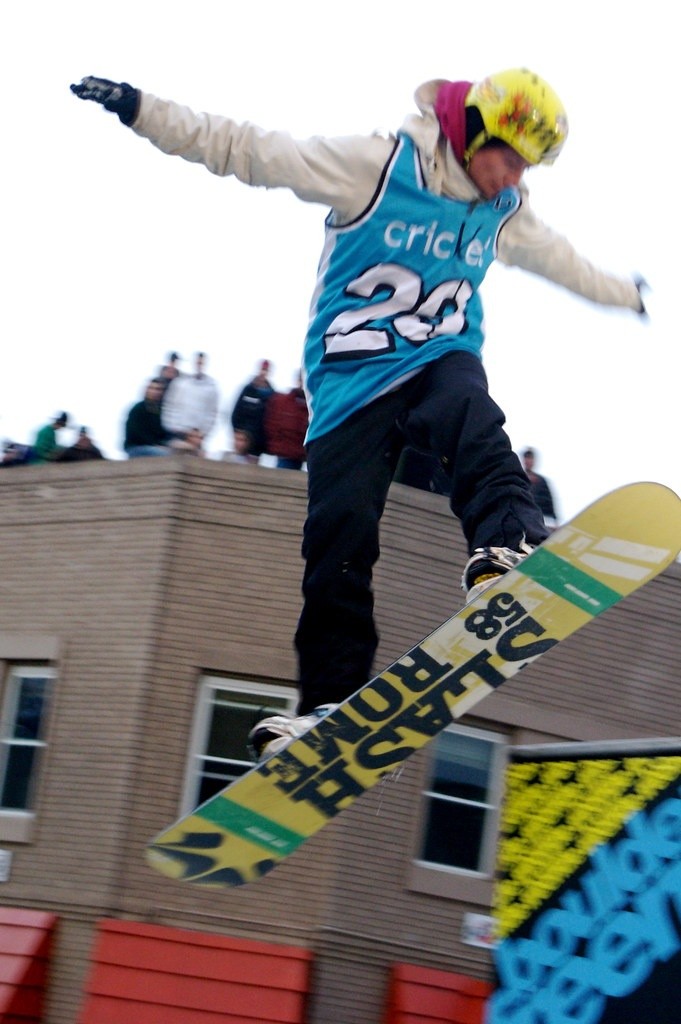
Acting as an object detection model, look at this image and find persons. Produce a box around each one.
[70,68,651,763]
[229,359,310,470]
[123,351,219,458]
[517,446,559,535]
[35,412,107,463]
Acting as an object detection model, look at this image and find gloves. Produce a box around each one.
[70,75,142,127]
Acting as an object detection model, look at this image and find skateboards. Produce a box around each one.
[143,480,681,892]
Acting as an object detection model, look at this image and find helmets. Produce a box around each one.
[465,69,568,166]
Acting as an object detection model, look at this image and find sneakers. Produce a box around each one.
[460,538,537,595]
[247,703,340,763]
